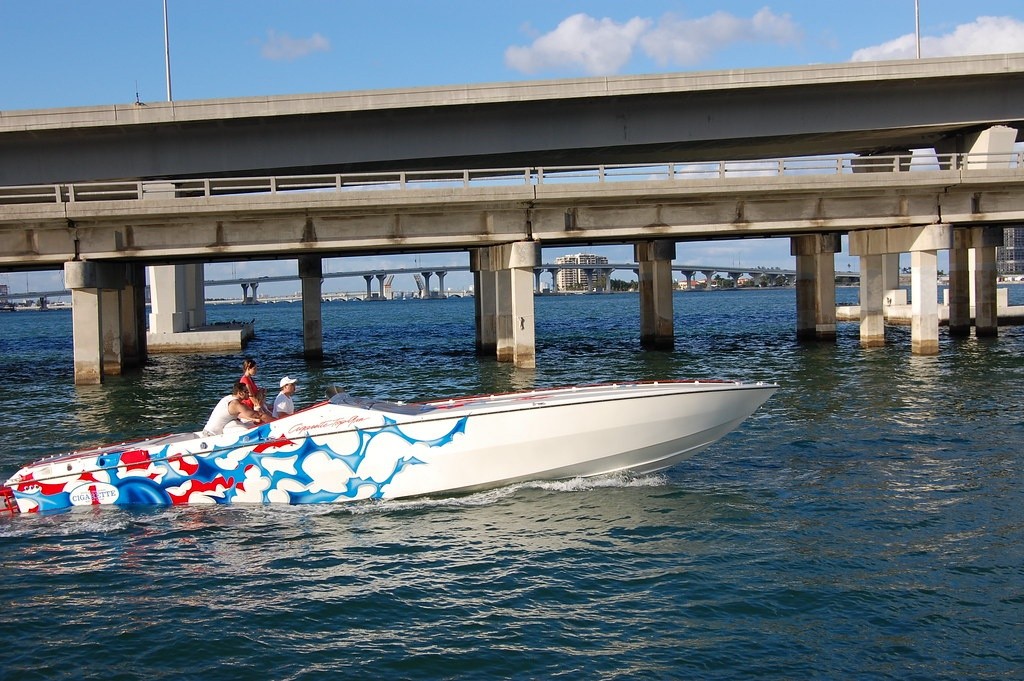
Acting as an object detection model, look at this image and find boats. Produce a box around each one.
[0,379,781,517]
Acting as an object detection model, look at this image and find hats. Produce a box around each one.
[280,376,298,388]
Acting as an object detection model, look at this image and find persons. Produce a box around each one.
[272,377,297,418]
[202,360,274,436]
